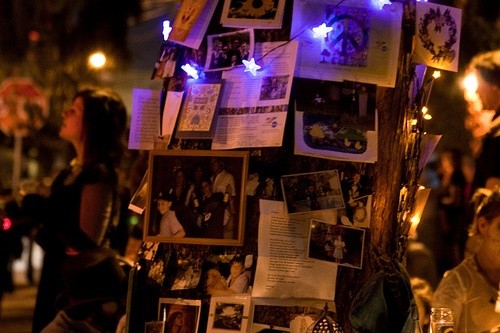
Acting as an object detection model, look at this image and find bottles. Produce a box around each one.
[429,308,454,333]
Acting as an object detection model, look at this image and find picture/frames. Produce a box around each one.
[143,149,249,245]
[206,296,254,333]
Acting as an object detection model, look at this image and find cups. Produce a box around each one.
[11,178,38,206]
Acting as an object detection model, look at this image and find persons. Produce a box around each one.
[322,233,348,262]
[341,171,364,220]
[307,120,366,150]
[163,50,177,78]
[154,155,239,240]
[404,48,499,333]
[209,258,249,295]
[165,311,184,333]
[0,187,25,293]
[289,174,332,210]
[21,88,130,333]
[210,38,251,69]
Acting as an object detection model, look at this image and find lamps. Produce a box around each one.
[307,304,344,333]
